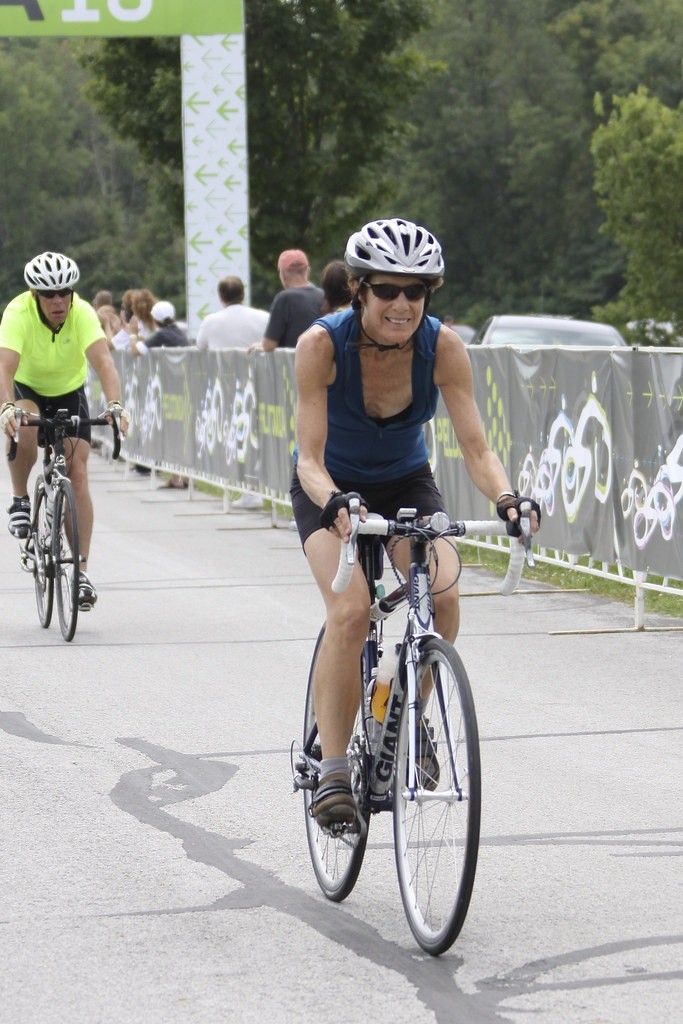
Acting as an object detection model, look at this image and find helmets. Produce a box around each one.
[343,218,445,287]
[22,250,80,290]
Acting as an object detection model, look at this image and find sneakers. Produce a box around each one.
[72,572,98,605]
[412,716,440,792]
[6,496,30,539]
[310,772,356,826]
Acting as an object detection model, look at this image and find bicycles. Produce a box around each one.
[5,404,124,643]
[293,499,538,957]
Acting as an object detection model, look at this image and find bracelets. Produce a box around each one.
[129,335,137,339]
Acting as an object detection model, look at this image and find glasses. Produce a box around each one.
[360,280,429,302]
[37,289,72,297]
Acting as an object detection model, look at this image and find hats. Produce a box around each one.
[277,250,308,272]
[151,301,176,323]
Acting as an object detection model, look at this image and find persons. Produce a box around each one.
[92,288,194,488]
[0,252,129,609]
[196,275,271,510]
[289,218,541,827]
[444,315,455,329]
[320,260,354,316]
[247,250,327,533]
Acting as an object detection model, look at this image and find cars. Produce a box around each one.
[465,315,630,349]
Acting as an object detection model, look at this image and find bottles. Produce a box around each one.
[371,641,403,726]
